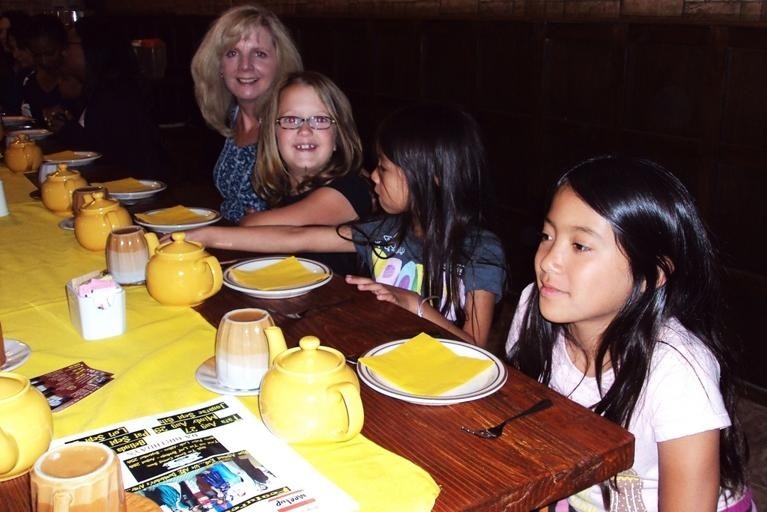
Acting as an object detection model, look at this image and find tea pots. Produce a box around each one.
[258,326,365,449]
[4,134,43,174]
[0,370,54,483]
[144,232,223,309]
[41,164,88,217]
[73,192,133,252]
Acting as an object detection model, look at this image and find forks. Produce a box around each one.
[460,399,553,439]
[265,297,356,320]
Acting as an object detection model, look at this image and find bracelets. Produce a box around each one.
[417,296,425,316]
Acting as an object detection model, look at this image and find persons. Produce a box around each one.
[238,71,371,277]
[1,0,151,162]
[504,152,758,512]
[160,101,511,349]
[192,3,304,224]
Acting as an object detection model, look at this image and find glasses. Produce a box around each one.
[275,114,337,131]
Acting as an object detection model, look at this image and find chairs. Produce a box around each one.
[487,291,520,353]
[726,378,767,512]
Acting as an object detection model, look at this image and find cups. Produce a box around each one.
[73,186,108,217]
[215,307,276,391]
[29,441,128,512]
[105,225,149,284]
[39,164,58,185]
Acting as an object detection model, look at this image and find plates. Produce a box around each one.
[58,217,74,230]
[109,179,168,199]
[194,355,260,396]
[222,256,334,300]
[134,206,224,234]
[29,189,41,200]
[2,116,103,167]
[1,339,31,371]
[126,492,163,512]
[356,338,509,406]
